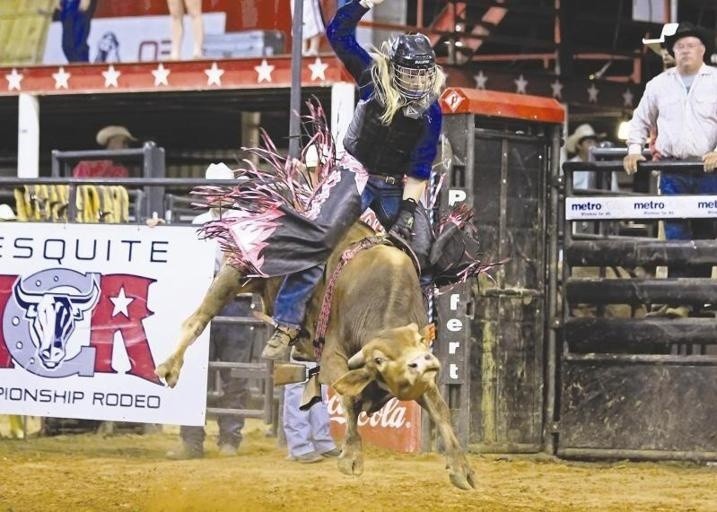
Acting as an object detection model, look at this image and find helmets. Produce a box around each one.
[388,33,438,103]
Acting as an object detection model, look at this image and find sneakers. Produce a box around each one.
[219,444,236,458]
[293,447,341,463]
[644,303,716,319]
[262,326,300,360]
[166,441,203,459]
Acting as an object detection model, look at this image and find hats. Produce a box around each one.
[642,23,717,62]
[96,125,139,147]
[565,124,607,154]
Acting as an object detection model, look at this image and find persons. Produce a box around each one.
[72,126,138,177]
[146,162,254,460]
[642,23,679,317]
[623,22,717,318]
[259,0,447,361]
[566,125,621,236]
[162,0,204,61]
[282,345,342,463]
[58,0,97,63]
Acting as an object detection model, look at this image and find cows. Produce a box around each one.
[154,166,479,491]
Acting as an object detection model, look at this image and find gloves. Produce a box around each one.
[390,208,415,249]
